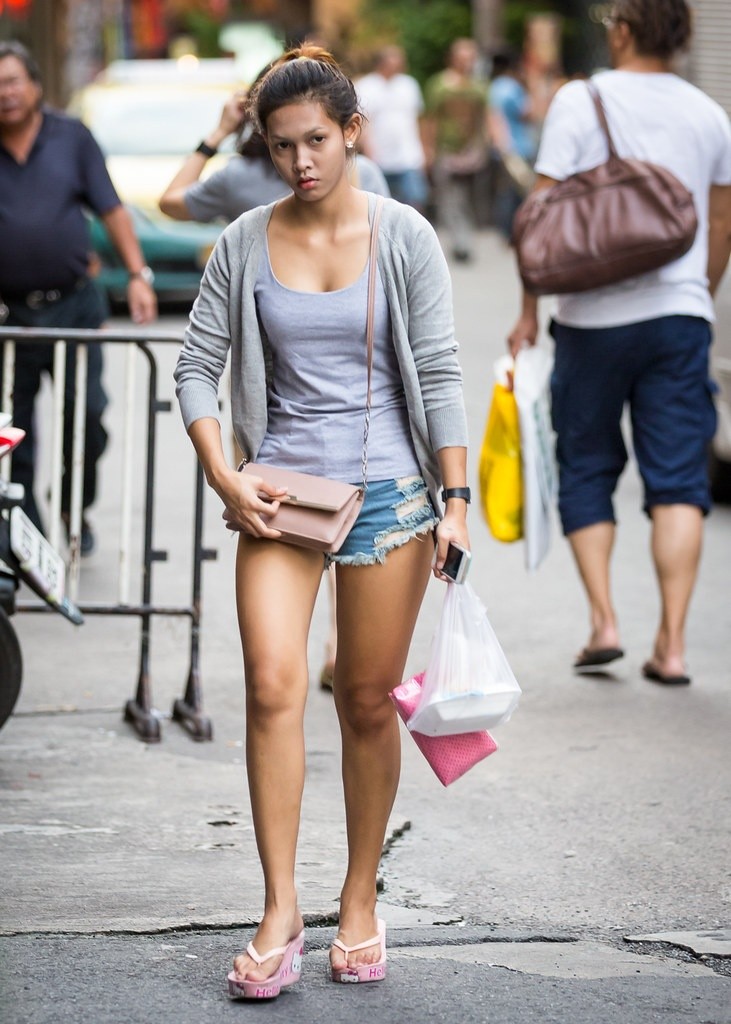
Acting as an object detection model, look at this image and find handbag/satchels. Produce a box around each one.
[388,670,498,787]
[222,460,364,554]
[482,329,561,564]
[406,581,523,737]
[510,79,697,296]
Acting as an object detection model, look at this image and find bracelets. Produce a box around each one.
[442,487,471,504]
[196,141,216,157]
[130,265,155,284]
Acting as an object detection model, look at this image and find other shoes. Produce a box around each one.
[62,515,95,555]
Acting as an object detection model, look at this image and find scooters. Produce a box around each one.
[0,414,85,729]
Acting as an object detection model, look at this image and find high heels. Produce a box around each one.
[227,930,305,999]
[331,917,387,983]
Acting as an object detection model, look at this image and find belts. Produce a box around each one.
[0,274,88,309]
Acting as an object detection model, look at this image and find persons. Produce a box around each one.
[0,41,158,555]
[509,0,731,684]
[157,11,588,245]
[157,46,471,998]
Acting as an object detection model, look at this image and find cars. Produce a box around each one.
[66,66,271,310]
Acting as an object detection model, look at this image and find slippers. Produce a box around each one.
[573,649,623,674]
[642,661,691,684]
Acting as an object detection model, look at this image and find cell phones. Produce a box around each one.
[438,541,471,584]
